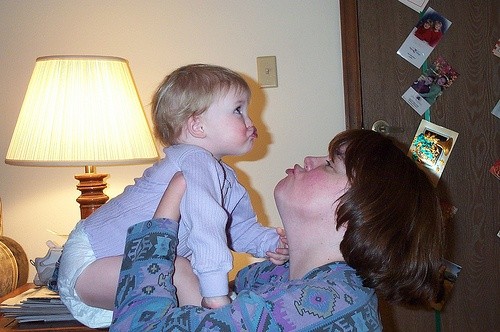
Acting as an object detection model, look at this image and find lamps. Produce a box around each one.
[5,55,160,222]
